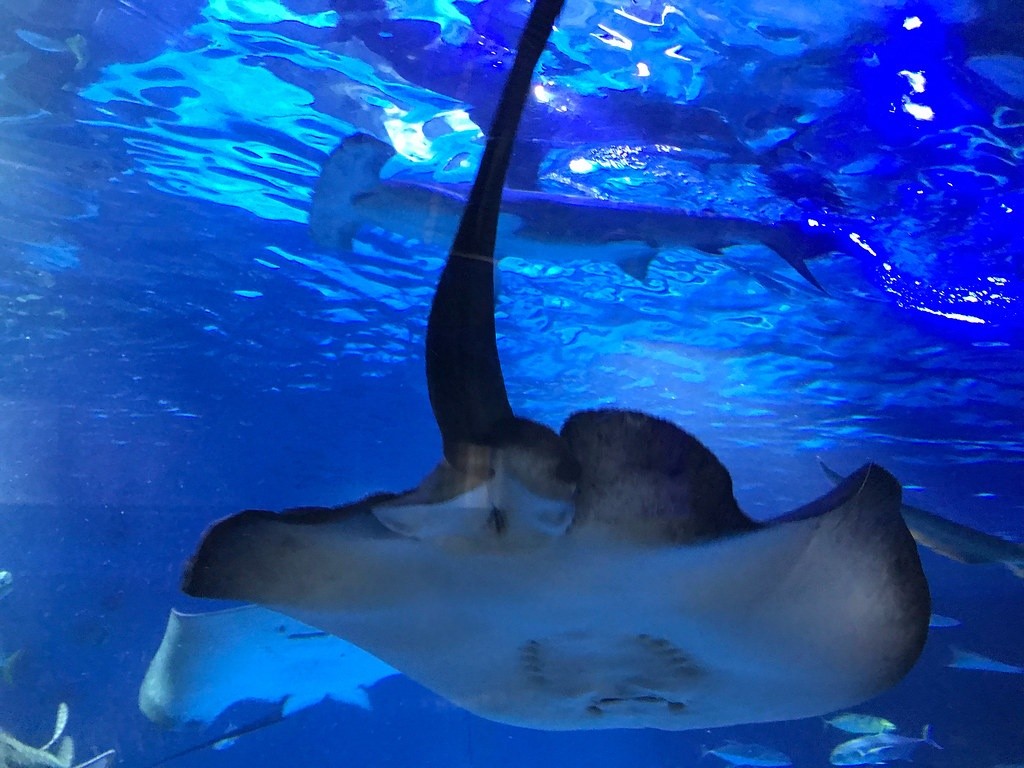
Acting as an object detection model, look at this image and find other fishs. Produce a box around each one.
[0,566,120,767]
[136,1,1023,768]
